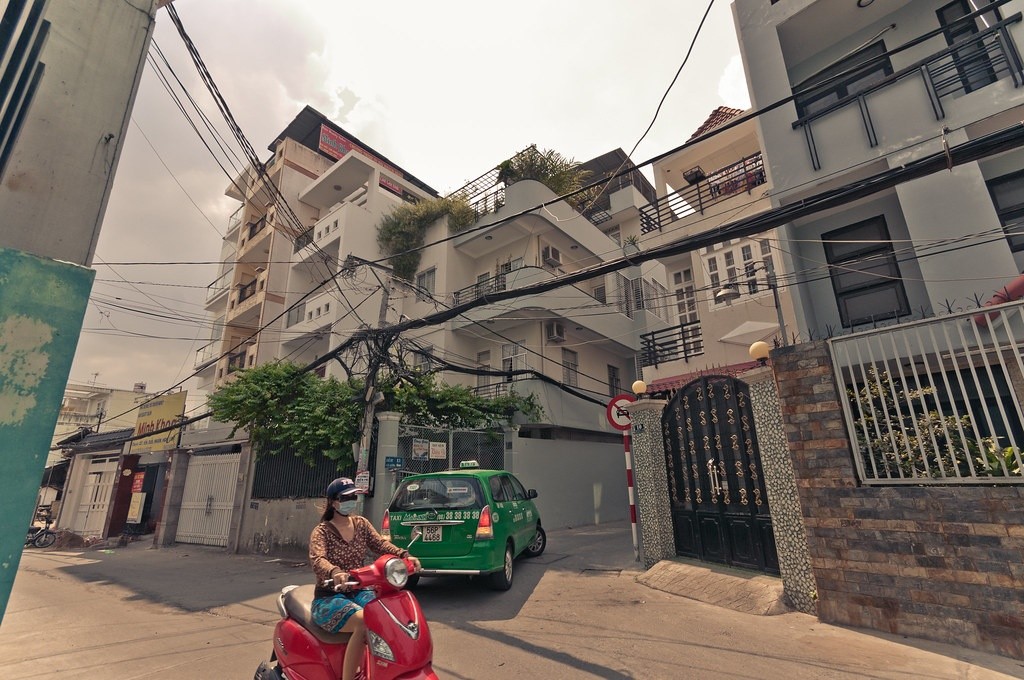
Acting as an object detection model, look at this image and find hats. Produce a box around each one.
[326,477,363,496]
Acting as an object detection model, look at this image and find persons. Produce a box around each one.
[308,477,421,679]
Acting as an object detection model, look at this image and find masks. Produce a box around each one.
[337,500,359,515]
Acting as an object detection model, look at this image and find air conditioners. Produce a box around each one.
[545,245,563,266]
[547,322,567,342]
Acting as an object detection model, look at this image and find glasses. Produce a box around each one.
[333,495,358,501]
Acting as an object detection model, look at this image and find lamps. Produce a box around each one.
[632,380,647,399]
[749,341,771,367]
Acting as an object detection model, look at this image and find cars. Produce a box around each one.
[378,460,548,592]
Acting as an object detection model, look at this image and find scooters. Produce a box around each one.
[254,523,438,680]
[22,511,57,549]
